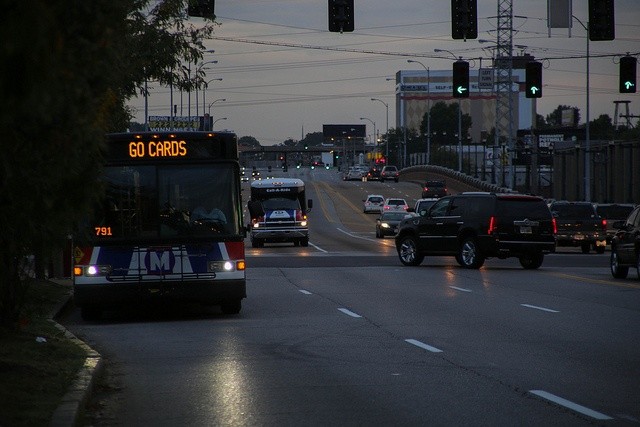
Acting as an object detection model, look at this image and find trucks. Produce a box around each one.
[248,178,312,247]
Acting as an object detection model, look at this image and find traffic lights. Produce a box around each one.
[526,62,542,98]
[453,62,469,98]
[620,57,637,93]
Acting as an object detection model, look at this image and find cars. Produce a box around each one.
[380,198,409,214]
[422,181,447,198]
[347,170,362,179]
[376,212,408,238]
[362,195,384,214]
[366,168,380,181]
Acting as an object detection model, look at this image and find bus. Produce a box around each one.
[72,132,247,324]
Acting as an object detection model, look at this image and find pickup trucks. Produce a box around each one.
[548,202,608,254]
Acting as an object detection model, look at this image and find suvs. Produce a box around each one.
[407,198,440,215]
[592,204,637,250]
[395,192,558,269]
[610,205,640,279]
[380,166,399,182]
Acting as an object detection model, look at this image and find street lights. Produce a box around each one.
[408,60,430,164]
[189,50,214,132]
[371,99,388,166]
[203,79,223,131]
[360,118,375,167]
[209,99,226,131]
[386,78,406,167]
[434,49,462,171]
[196,61,217,131]
